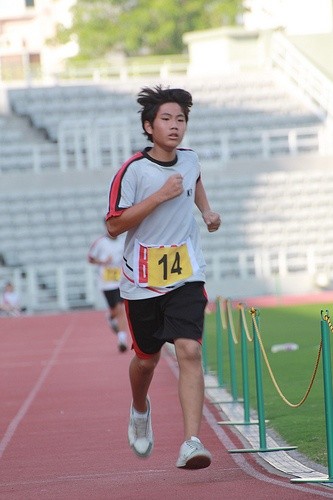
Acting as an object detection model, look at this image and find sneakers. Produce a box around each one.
[127,394,154,460]
[174,435,212,470]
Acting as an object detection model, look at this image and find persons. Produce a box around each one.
[88,210,130,352]
[105,84,222,469]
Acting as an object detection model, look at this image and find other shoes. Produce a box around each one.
[117,342,127,352]
[107,315,120,333]
